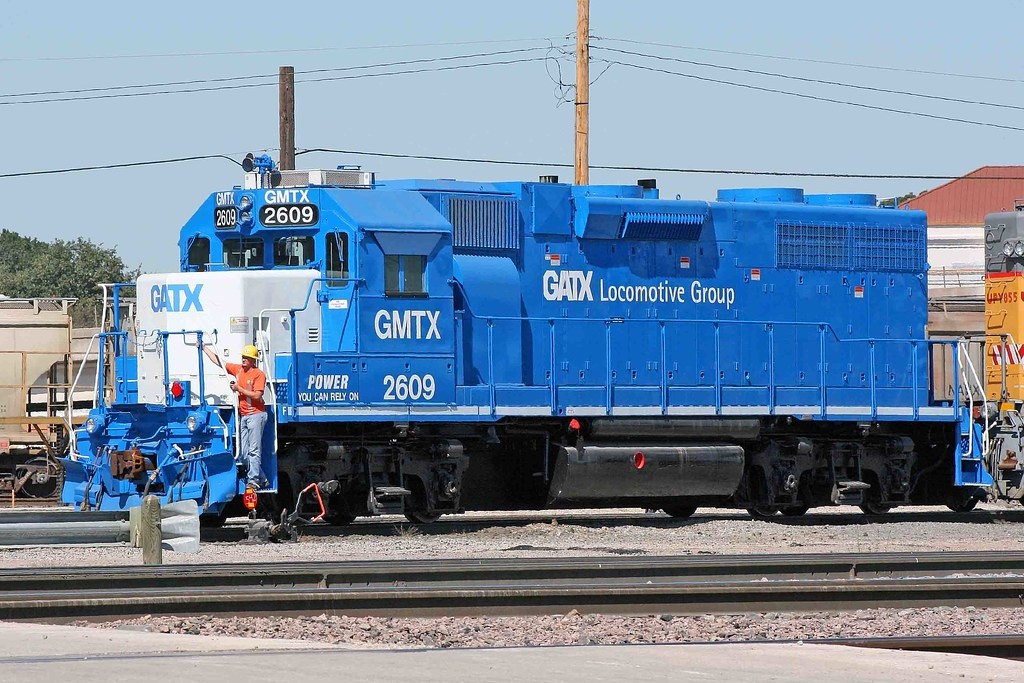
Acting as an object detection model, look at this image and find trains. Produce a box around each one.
[0,296,136,499]
[54,148,1024,528]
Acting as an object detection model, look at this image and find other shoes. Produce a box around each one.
[246,483,257,490]
[260,481,270,488]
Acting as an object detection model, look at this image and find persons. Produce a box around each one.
[195,340,270,491]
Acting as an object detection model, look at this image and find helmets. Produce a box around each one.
[241,345,260,359]
